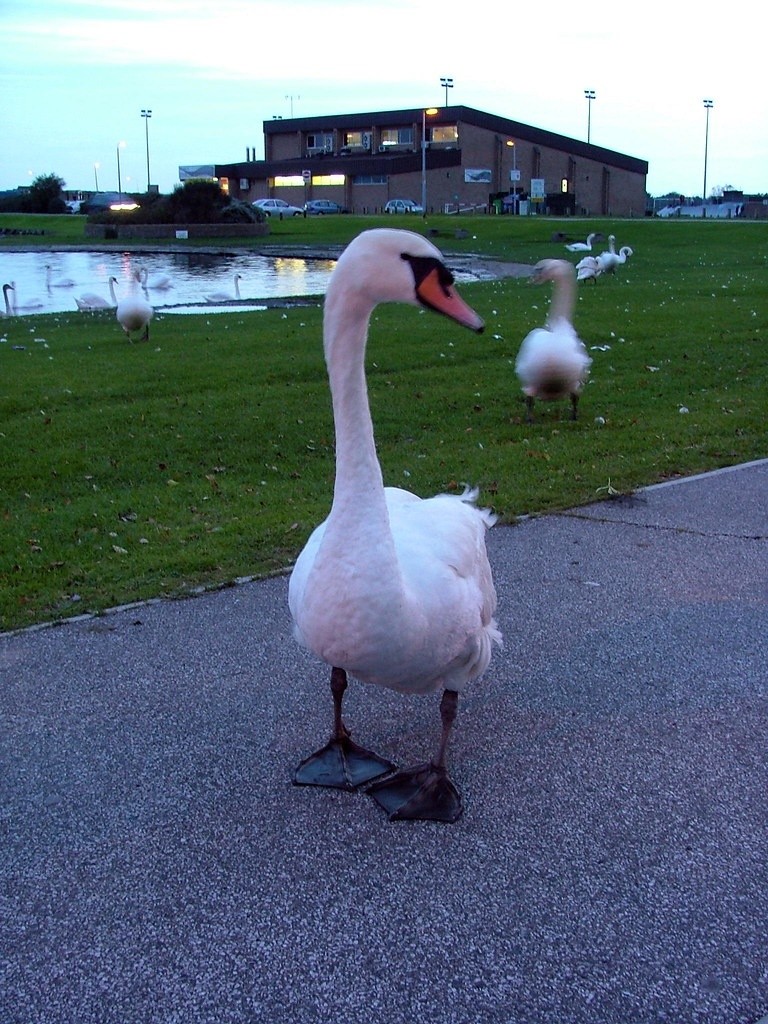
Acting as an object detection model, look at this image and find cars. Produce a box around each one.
[384,199,422,215]
[305,199,349,215]
[65,192,140,215]
[254,199,303,217]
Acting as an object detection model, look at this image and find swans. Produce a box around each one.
[564,232,636,286]
[3,254,243,347]
[514,260,592,422]
[287,228,501,823]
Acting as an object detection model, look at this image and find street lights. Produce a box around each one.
[440,78,454,108]
[140,109,152,191]
[507,141,517,215]
[117,140,127,210]
[584,90,596,143]
[94,161,102,194]
[703,99,713,203]
[422,108,438,210]
